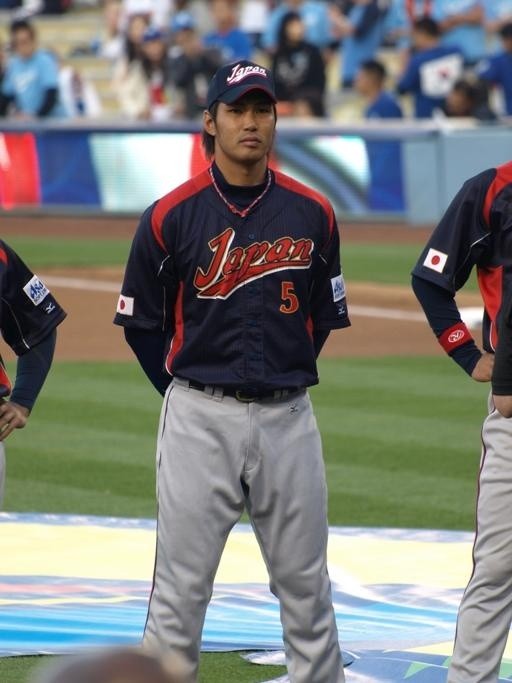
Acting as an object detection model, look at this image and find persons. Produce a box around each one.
[407,159,512,683]
[0,238,70,443]
[112,59,354,683]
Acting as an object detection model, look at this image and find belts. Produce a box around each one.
[188,379,306,402]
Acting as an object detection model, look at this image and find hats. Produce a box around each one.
[207,61,277,110]
